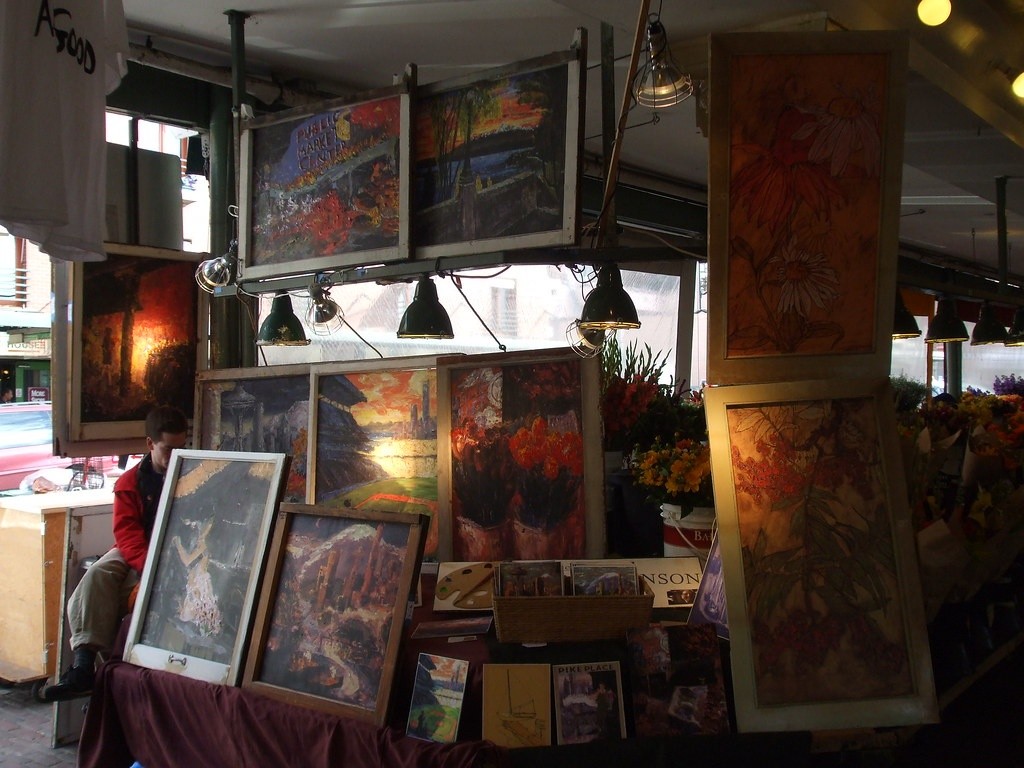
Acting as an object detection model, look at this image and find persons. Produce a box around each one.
[42,404,196,696]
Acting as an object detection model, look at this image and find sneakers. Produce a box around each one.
[43,665,97,701]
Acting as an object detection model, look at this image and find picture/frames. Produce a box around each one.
[384,25,588,267]
[192,363,310,505]
[433,342,609,564]
[706,29,910,387]
[236,61,418,282]
[241,502,431,729]
[122,448,295,686]
[65,241,211,444]
[702,372,942,731]
[305,354,464,574]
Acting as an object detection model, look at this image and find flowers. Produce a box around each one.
[601,332,1024,543]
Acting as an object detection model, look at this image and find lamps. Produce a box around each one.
[991,57,1024,98]
[1004,308,1024,347]
[255,290,312,347]
[574,254,641,330]
[396,278,455,340]
[194,253,235,294]
[892,287,922,339]
[632,22,694,109]
[303,280,345,336]
[970,302,1011,346]
[924,294,970,344]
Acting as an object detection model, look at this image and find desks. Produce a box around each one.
[75,649,1024,768]
[0,488,115,703]
